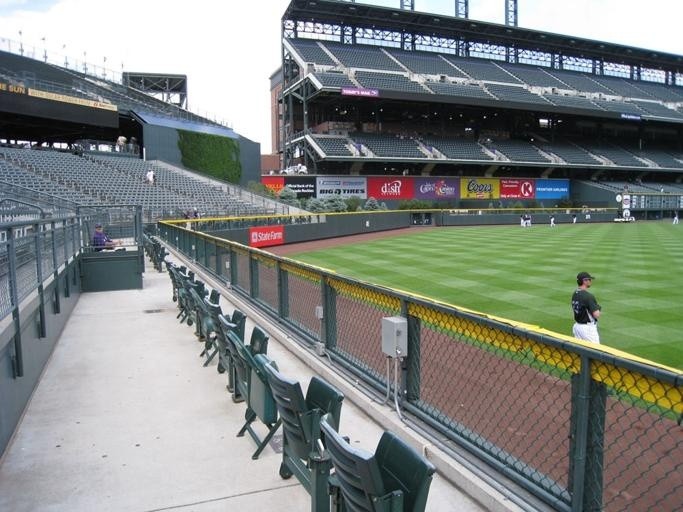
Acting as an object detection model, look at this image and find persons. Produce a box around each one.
[571,211,577,224]
[185,207,199,229]
[146,169,154,187]
[548,212,556,227]
[628,176,664,195]
[572,271,601,344]
[116,132,138,153]
[520,212,533,227]
[90,222,126,252]
[672,207,679,225]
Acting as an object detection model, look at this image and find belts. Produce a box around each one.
[577,321,596,325]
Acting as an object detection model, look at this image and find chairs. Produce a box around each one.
[308,418,436,512]
[144,233,297,461]
[254,353,345,496]
[2,145,288,272]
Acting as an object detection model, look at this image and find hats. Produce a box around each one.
[577,271,596,280]
[95,223,102,227]
